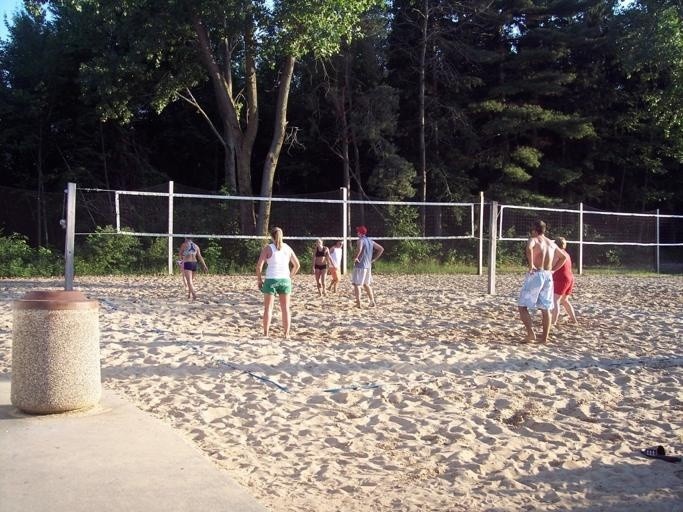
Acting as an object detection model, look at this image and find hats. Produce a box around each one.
[355,225,367,233]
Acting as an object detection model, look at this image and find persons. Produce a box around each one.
[177,257,188,289]
[550,236,579,326]
[326,240,344,294]
[312,238,338,298]
[517,219,567,345]
[179,232,209,301]
[255,226,301,341]
[351,226,384,308]
